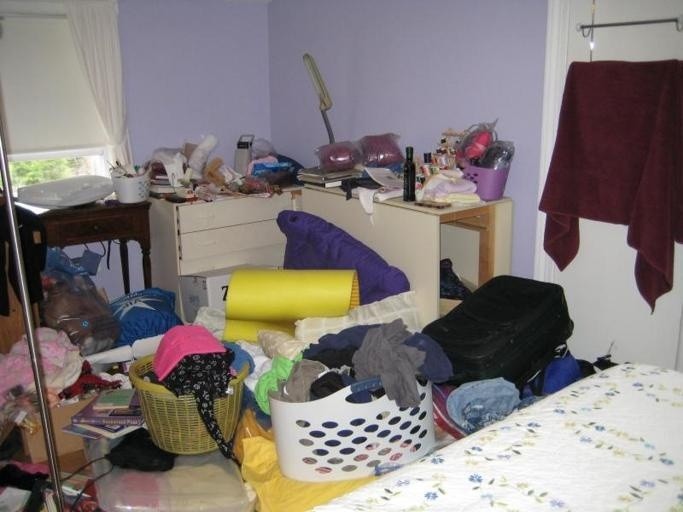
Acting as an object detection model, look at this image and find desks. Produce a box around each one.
[13,196,157,302]
[296,175,516,334]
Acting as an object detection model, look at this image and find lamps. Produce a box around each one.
[303,53,341,144]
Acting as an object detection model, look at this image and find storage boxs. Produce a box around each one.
[429,391,468,446]
[177,265,277,326]
[22,397,117,485]
[80,411,255,510]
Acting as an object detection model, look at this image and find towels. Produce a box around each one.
[538,60,683,318]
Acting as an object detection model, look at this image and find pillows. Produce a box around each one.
[276,209,413,305]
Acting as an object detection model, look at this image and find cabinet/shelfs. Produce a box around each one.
[142,178,304,326]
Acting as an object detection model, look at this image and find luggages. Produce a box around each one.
[421,276,574,390]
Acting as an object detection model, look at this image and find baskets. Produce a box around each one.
[457,157,510,200]
[129,352,249,455]
[109,166,152,204]
[268,371,434,483]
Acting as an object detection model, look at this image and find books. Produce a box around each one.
[71,421,142,439]
[414,199,450,209]
[91,388,134,409]
[70,398,145,425]
[296,166,358,180]
[60,425,103,439]
[59,472,88,496]
[364,166,405,188]
[0,485,31,511]
[302,180,342,189]
[149,165,192,198]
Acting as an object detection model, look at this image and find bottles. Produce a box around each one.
[403,146,415,203]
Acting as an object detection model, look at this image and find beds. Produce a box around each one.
[307,361,682,512]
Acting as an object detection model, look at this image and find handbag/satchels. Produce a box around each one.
[104,428,178,471]
